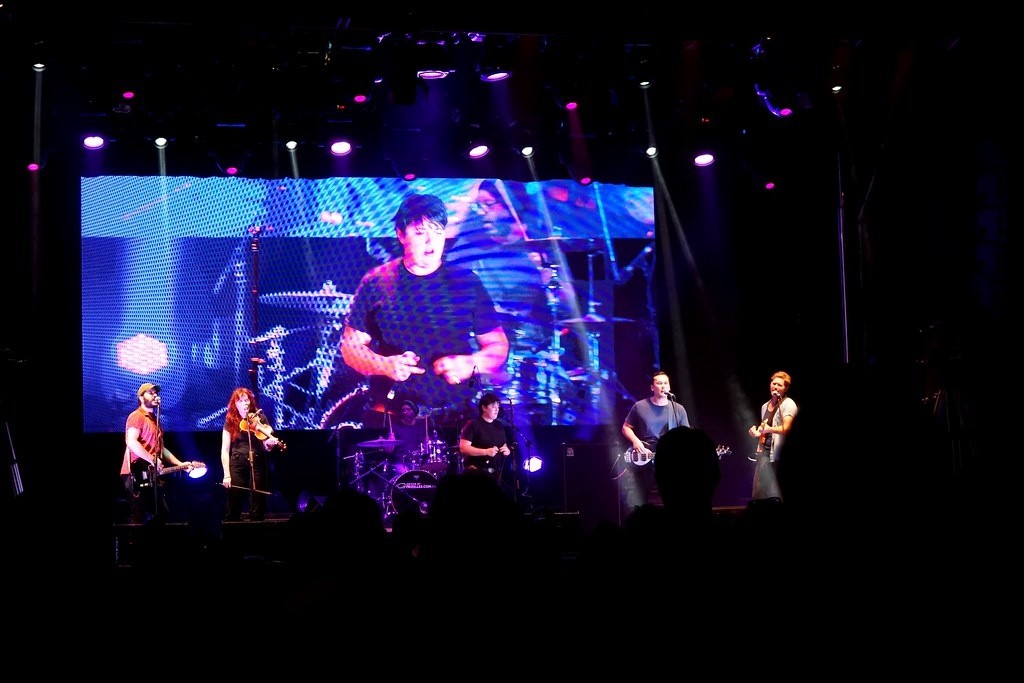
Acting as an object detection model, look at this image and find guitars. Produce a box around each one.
[756,418,767,454]
[130,457,208,507]
[620,441,733,473]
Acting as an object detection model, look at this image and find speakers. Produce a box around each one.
[537,442,623,527]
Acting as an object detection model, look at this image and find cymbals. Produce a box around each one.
[502,235,605,254]
[448,445,459,448]
[551,314,636,330]
[356,439,404,447]
[257,290,354,314]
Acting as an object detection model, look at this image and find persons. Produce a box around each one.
[342,194,509,426]
[120,382,193,522]
[221,388,278,521]
[0,389,1023,682]
[749,372,797,497]
[449,179,575,310]
[622,372,691,506]
[460,394,511,482]
[392,400,424,442]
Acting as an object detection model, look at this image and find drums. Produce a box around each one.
[320,386,371,428]
[496,354,549,408]
[408,449,421,467]
[419,440,448,474]
[385,469,438,517]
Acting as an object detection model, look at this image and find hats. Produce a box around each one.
[138,383,161,397]
[405,400,419,414]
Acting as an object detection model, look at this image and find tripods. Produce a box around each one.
[568,323,636,424]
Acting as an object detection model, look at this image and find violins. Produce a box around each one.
[239,412,288,453]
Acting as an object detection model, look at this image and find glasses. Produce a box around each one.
[401,409,412,412]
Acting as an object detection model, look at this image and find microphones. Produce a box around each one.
[469,366,475,388]
[155,396,161,403]
[248,226,274,235]
[422,503,428,509]
[243,404,248,412]
[358,383,364,395]
[771,390,781,398]
[663,391,674,397]
[388,351,417,400]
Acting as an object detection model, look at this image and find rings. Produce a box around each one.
[454,376,460,385]
